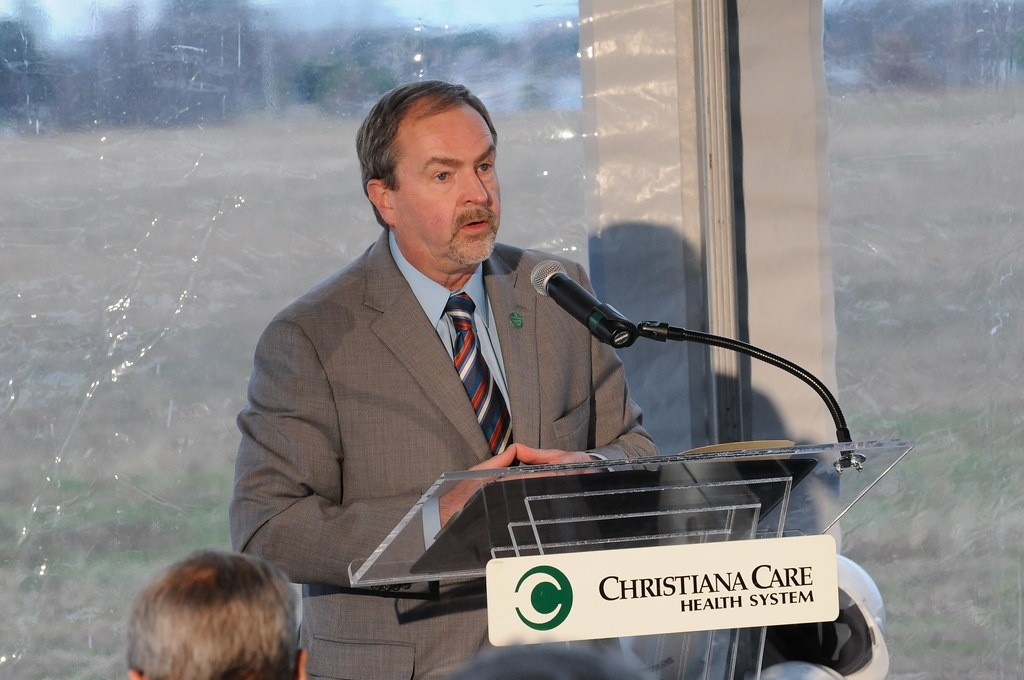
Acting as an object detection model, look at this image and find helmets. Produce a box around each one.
[757,554,889,680]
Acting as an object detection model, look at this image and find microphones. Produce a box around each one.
[529,260,634,349]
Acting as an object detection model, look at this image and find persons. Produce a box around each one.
[128,548,307,680]
[230,81,656,680]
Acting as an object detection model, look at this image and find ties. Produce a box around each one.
[442,291,520,468]
